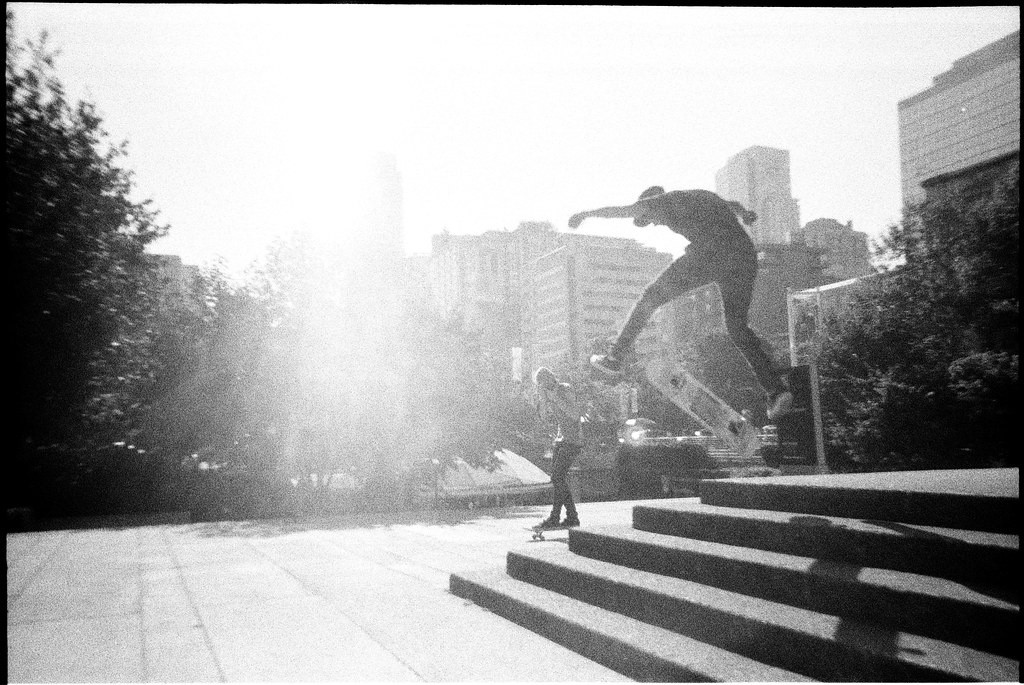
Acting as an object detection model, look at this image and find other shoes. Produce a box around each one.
[532,517,562,530]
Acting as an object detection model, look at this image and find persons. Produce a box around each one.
[566,185,794,419]
[532,365,581,529]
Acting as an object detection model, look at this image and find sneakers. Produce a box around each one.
[590,354,620,375]
[559,515,580,528]
[765,386,793,419]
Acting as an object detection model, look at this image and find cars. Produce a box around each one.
[622,417,671,444]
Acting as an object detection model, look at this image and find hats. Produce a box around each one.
[634,187,664,227]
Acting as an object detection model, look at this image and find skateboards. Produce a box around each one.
[521,521,584,540]
[605,335,763,459]
